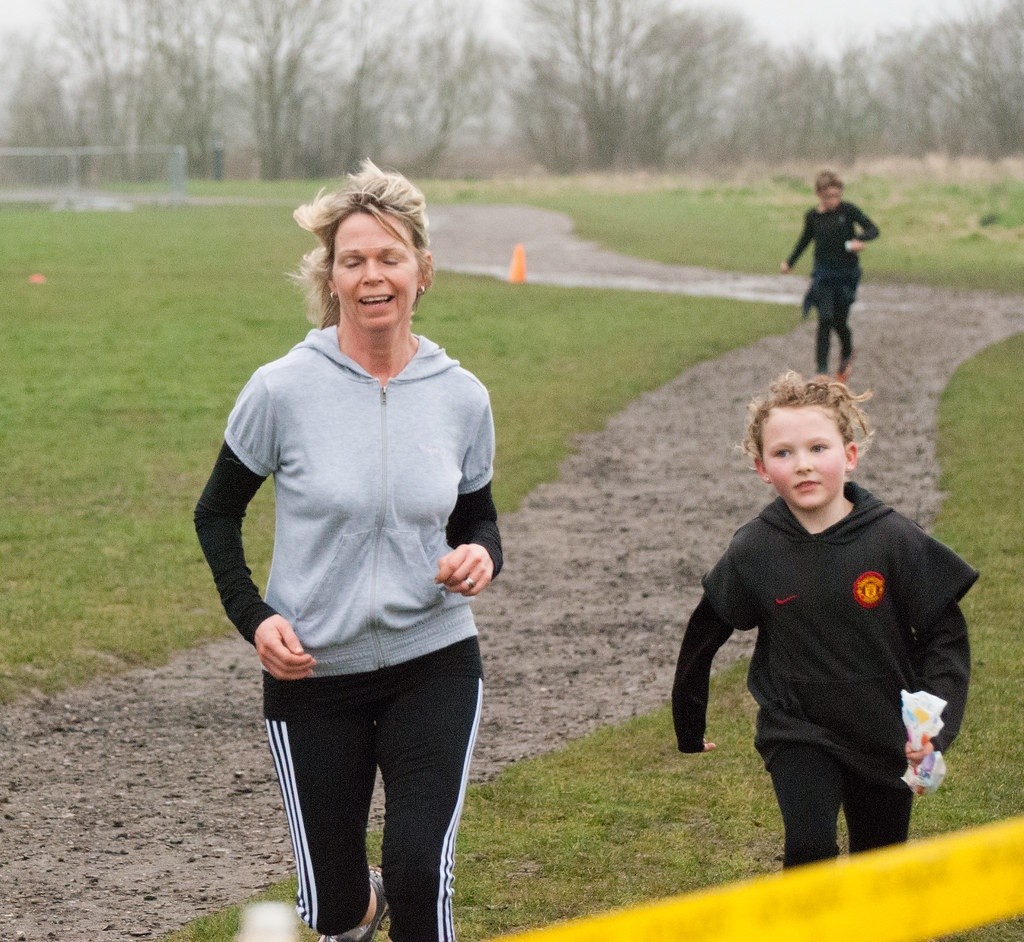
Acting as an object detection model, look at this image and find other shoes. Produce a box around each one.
[835,353,855,382]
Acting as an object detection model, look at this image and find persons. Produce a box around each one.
[193,156,504,942]
[671,374,981,875]
[780,172,881,385]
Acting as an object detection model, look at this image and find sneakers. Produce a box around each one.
[317,864,392,942]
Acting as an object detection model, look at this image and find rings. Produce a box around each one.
[464,577,475,588]
